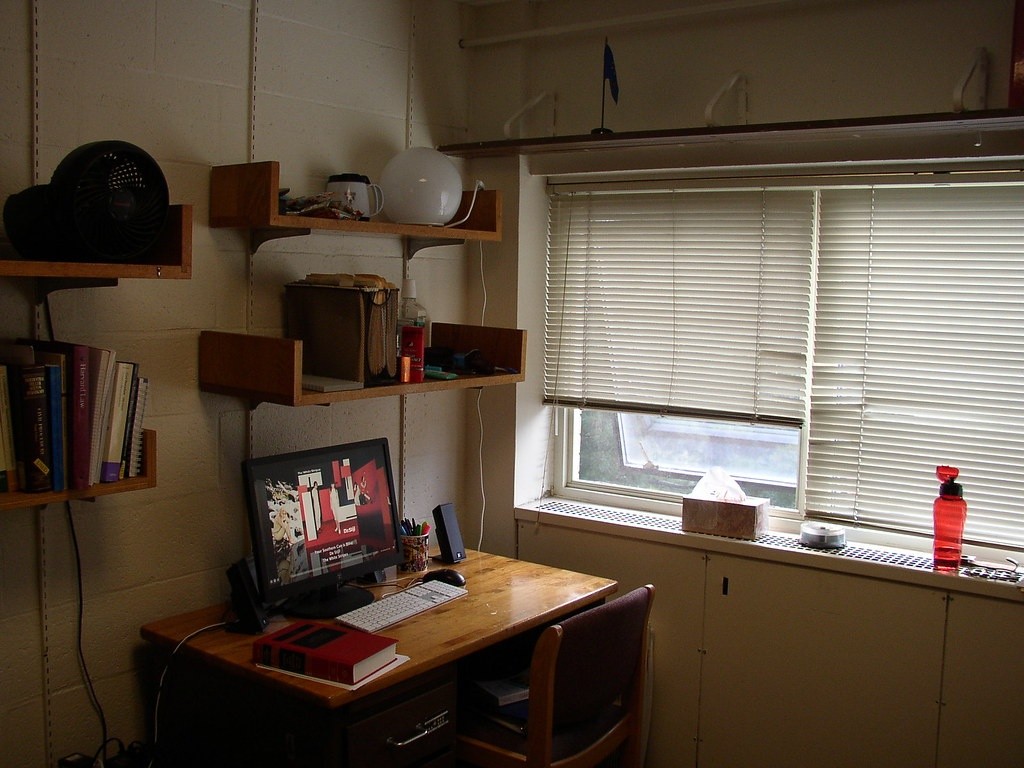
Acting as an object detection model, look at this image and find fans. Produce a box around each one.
[4,141,171,260]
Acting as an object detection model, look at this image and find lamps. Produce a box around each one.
[379,146,462,226]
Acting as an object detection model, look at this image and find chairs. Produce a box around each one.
[458,585,656,768]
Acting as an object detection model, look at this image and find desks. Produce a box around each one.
[141,542,620,768]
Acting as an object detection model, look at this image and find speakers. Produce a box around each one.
[224,556,270,635]
[430,503,467,564]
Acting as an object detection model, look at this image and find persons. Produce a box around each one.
[273,507,297,545]
[353,476,373,506]
[329,482,342,534]
[312,481,324,533]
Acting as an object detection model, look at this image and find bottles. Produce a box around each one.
[932,465,968,571]
[397,279,427,357]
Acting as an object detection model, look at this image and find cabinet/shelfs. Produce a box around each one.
[197,161,530,414]
[0,198,194,511]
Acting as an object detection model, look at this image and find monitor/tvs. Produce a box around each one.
[240,436,405,619]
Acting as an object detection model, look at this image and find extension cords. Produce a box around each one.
[102,750,148,768]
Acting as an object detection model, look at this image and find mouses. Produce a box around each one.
[423,569,466,587]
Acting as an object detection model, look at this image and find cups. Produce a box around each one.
[398,533,430,573]
[326,173,385,222]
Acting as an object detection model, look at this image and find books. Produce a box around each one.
[0,335,150,493]
[288,277,399,391]
[481,673,529,706]
[252,620,400,685]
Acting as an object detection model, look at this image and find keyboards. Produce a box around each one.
[335,580,468,634]
